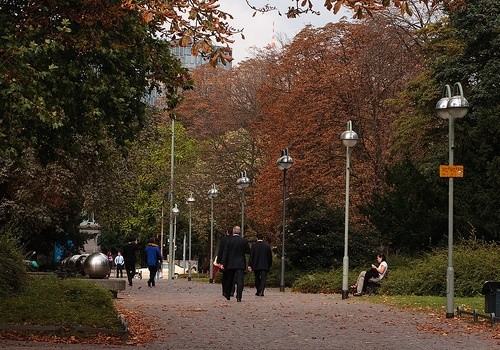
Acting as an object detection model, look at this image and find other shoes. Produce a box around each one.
[354,292,362,296]
[256,293,264,296]
[152,285,155,286]
[148,281,151,287]
[129,273,131,280]
[231,292,234,296]
[130,282,132,286]
[350,285,357,289]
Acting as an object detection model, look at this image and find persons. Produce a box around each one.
[349,254,388,296]
[107,251,113,277]
[213,226,250,302]
[114,252,124,278]
[120,237,139,286]
[247,234,272,297]
[144,237,163,287]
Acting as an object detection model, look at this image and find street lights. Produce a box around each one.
[276,148,294,292]
[434,82,470,319]
[235,170,250,238]
[339,120,359,300]
[171,203,180,280]
[206,183,220,284]
[186,193,196,281]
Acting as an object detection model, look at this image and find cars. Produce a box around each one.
[133,262,188,280]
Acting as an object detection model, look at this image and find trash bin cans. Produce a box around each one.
[481,281,500,322]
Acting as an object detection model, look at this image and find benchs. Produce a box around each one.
[350,283,382,296]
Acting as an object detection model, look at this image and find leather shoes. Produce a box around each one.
[227,296,230,300]
[237,298,241,302]
